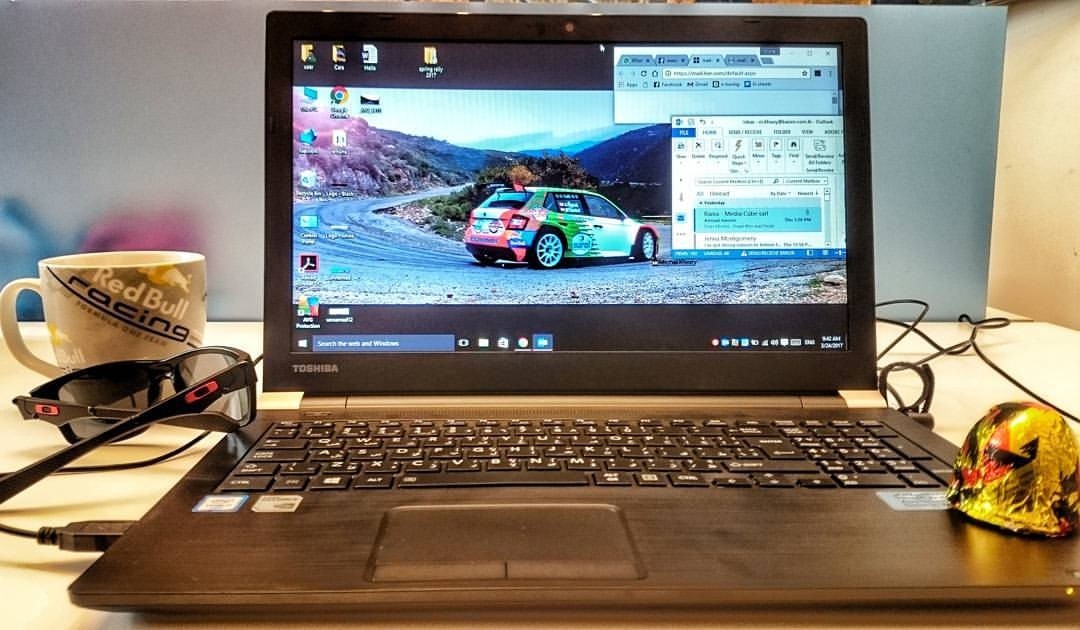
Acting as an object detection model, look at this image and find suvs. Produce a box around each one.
[461,185,662,271]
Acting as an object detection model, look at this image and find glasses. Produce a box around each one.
[0,346,257,502]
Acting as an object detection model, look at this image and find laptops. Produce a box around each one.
[67,8,1080,612]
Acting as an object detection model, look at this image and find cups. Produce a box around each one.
[0,250,209,412]
